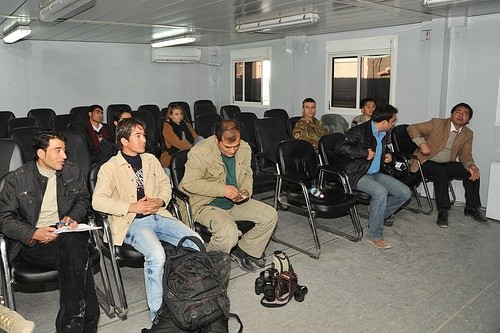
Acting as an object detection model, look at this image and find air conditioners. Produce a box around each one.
[150,47,201,63]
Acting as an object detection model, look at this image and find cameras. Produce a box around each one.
[255,268,308,303]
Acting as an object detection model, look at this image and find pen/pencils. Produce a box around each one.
[55,220,74,225]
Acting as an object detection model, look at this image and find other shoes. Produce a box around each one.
[464,206,490,223]
[437,211,449,227]
[366,237,392,249]
[232,245,255,272]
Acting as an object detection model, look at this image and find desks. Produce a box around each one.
[485,162,500,220]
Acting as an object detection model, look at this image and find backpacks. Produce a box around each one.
[141,234,244,333]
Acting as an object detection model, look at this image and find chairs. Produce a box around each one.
[0,100,457,319]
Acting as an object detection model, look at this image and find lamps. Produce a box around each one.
[152,26,196,40]
[39,0,96,22]
[2,26,31,44]
[424,0,486,9]
[151,34,196,48]
[234,2,317,25]
[234,13,320,32]
[2,18,35,34]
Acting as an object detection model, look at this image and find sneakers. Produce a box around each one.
[0,305,35,333]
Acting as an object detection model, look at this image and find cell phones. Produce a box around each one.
[235,193,248,203]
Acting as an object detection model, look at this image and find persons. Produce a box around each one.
[162,104,200,153]
[292,98,331,152]
[99,108,170,167]
[351,98,376,128]
[81,105,115,156]
[335,104,411,249]
[177,118,278,272]
[0,129,100,333]
[91,118,205,322]
[407,103,486,227]
